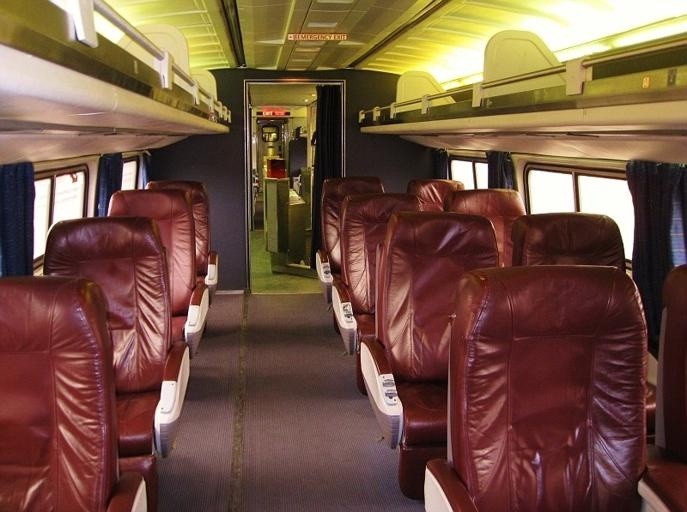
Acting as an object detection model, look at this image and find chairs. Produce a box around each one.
[314,174,684,511]
[1,180,219,511]
